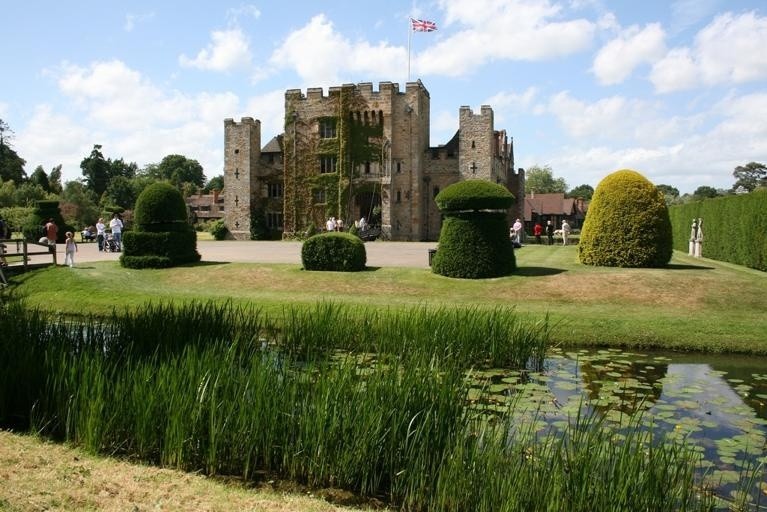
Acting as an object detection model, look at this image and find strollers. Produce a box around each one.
[102,230,121,251]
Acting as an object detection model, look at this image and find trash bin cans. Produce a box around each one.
[429,250,436,266]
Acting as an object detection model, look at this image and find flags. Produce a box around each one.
[410,18,438,33]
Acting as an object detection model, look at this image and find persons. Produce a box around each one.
[509,218,554,248]
[326,215,368,234]
[44,218,59,252]
[82,213,123,252]
[690,217,704,243]
[63,231,78,268]
[561,219,571,246]
